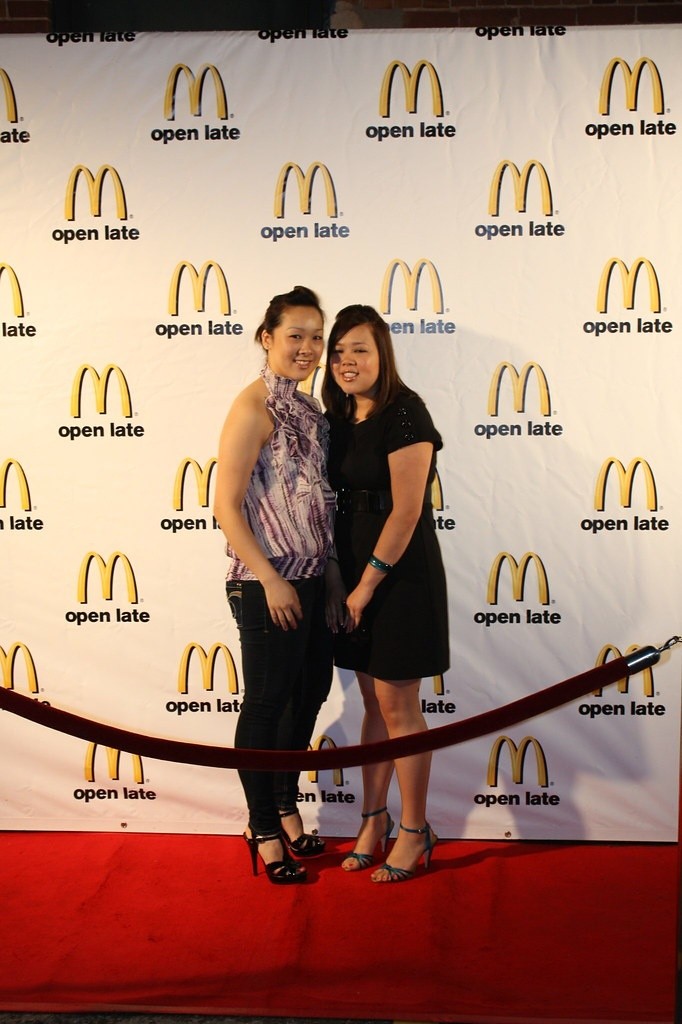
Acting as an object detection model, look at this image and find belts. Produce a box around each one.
[337,483,432,513]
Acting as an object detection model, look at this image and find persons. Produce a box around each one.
[214,285,329,885]
[321,304,450,882]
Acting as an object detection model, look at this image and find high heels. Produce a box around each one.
[371,823,438,883]
[341,807,394,871]
[244,823,307,883]
[279,809,326,856]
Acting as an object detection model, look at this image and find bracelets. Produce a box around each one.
[369,554,392,574]
[327,556,339,562]
